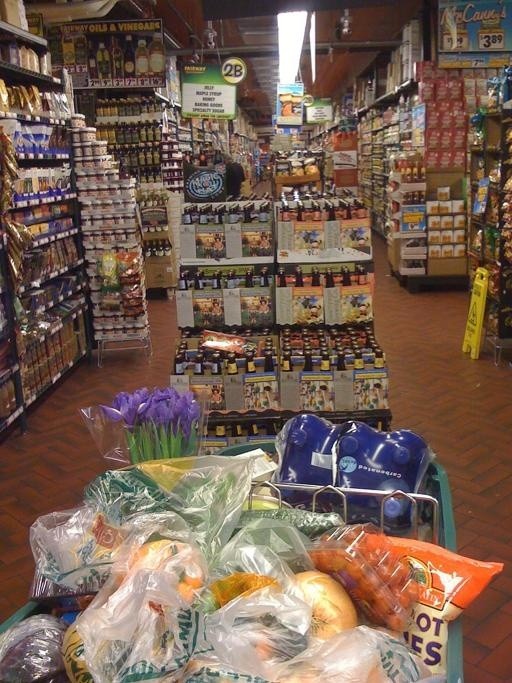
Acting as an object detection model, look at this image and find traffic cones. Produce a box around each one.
[462,267,489,359]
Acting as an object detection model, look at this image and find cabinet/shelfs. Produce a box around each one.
[356,80,415,236]
[89,93,194,297]
[3,20,91,400]
[464,65,512,366]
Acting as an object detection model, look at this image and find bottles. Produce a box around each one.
[109,35,123,78]
[178,273,187,290]
[195,272,203,290]
[85,244,127,256]
[358,267,366,285]
[95,120,161,142]
[108,142,160,166]
[82,199,134,211]
[87,40,97,79]
[246,271,253,287]
[77,184,135,198]
[73,127,97,143]
[135,40,147,75]
[73,141,107,156]
[124,35,135,77]
[226,272,235,288]
[93,312,135,322]
[76,169,119,182]
[147,32,164,73]
[280,270,286,286]
[89,259,96,269]
[174,326,274,375]
[71,113,85,127]
[74,156,108,169]
[94,324,144,334]
[260,270,268,287]
[212,272,220,289]
[295,269,304,287]
[343,268,351,286]
[183,201,270,226]
[96,96,155,116]
[282,198,364,222]
[326,270,334,288]
[282,326,384,373]
[82,228,137,242]
[312,270,320,286]
[131,167,161,183]
[80,214,135,227]
[97,42,110,79]
[144,239,171,256]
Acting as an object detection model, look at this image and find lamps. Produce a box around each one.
[275,11,307,86]
